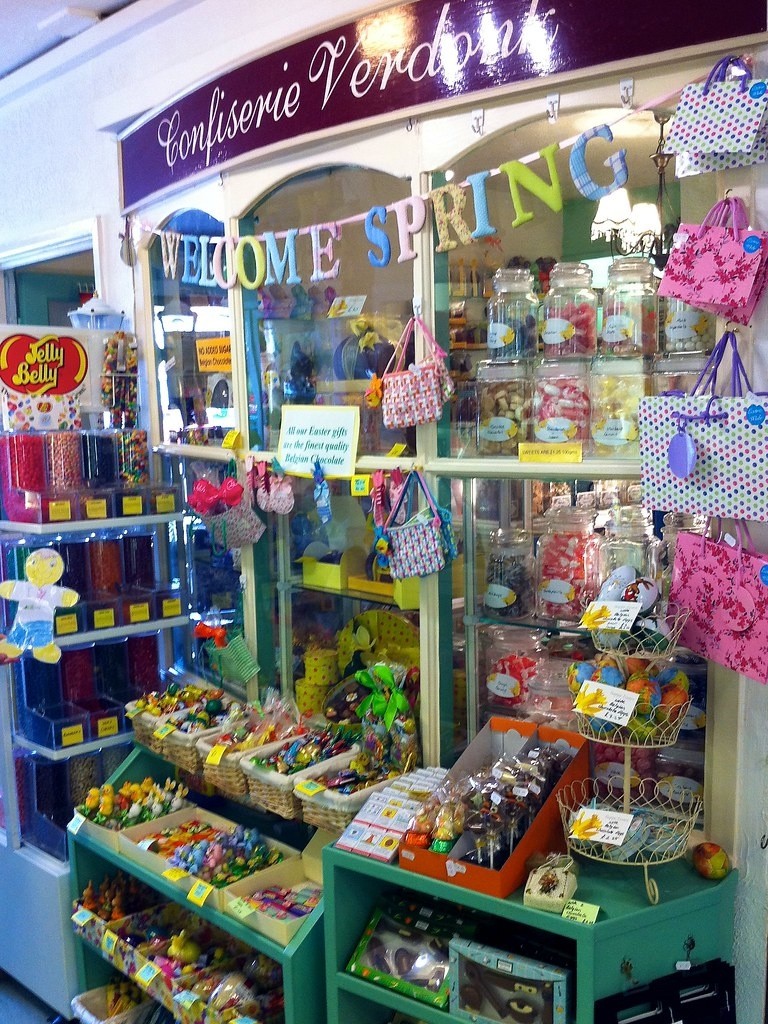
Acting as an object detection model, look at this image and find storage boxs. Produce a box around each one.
[73,716,592,1024]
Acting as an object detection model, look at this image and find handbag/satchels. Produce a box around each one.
[666,54,768,179]
[380,317,458,430]
[196,460,267,555]
[666,517,768,684]
[374,469,459,582]
[656,196,768,326]
[639,331,766,523]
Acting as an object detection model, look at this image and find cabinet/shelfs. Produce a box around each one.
[0,327,190,1021]
[66,740,328,1023]
[323,843,740,1024]
[262,318,713,831]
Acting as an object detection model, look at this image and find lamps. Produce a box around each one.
[68,290,126,331]
[588,108,680,271]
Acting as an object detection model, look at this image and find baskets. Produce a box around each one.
[128,693,403,838]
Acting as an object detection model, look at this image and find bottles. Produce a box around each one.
[485,527,535,621]
[487,625,704,816]
[602,257,658,354]
[544,262,597,358]
[600,505,665,583]
[538,507,600,620]
[659,512,713,616]
[101,337,138,428]
[475,367,713,462]
[487,268,538,359]
[665,295,715,352]
